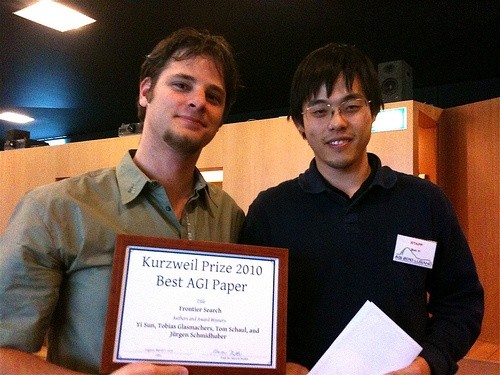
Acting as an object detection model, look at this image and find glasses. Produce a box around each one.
[300,98,373,121]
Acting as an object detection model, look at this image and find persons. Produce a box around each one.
[237,41,486,375]
[0,27,248,375]
